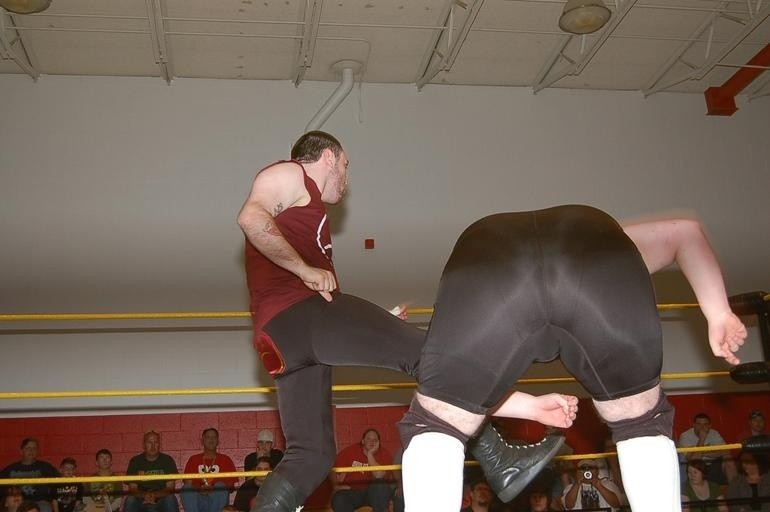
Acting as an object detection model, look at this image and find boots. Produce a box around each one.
[254,471,307,512]
[470,423,566,504]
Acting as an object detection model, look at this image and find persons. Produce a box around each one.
[395,203,748,512]
[235,129,568,512]
[1,429,239,511]
[235,412,770,512]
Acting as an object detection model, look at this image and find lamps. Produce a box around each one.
[0,0,51,14]
[559,0,612,35]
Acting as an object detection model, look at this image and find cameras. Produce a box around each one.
[579,462,593,481]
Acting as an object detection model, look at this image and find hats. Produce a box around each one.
[257,429,273,442]
